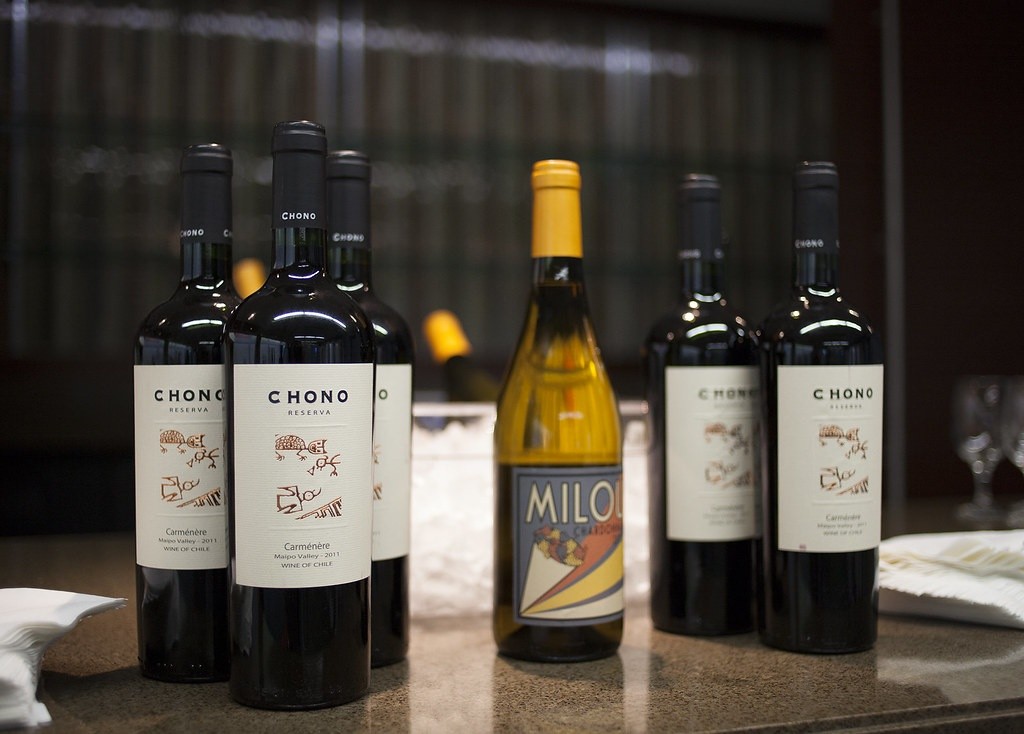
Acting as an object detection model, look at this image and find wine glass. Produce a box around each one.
[1000,385,1024,529]
[945,372,1014,521]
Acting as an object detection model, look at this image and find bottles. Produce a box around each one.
[228,116,378,714]
[124,138,246,689]
[316,146,415,669]
[641,168,767,639]
[419,306,498,400]
[491,159,627,663]
[768,159,886,653]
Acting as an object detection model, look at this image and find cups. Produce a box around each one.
[401,398,498,616]
[607,398,653,609]
